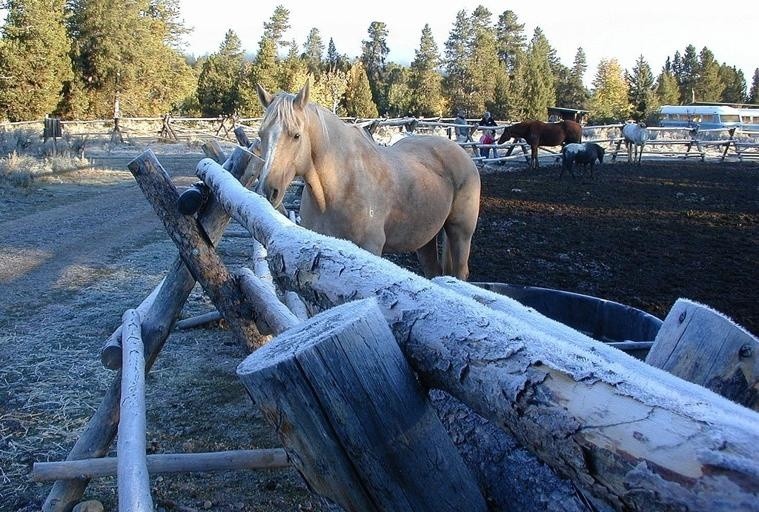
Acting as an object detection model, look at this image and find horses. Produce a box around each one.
[497,119,582,169]
[621,121,649,162]
[559,143,606,186]
[254,77,482,282]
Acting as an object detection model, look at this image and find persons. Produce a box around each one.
[477,110,501,164]
[454,110,469,142]
